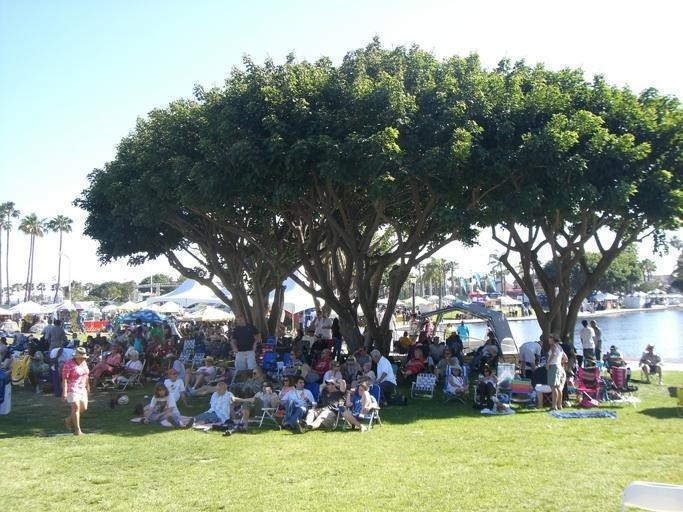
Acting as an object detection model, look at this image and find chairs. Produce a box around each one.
[81,339,382,432]
[443,352,659,408]
[409,372,437,400]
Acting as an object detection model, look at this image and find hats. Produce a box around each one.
[325,379,336,385]
[72,347,89,359]
[610,345,615,350]
[204,356,214,362]
[646,344,654,349]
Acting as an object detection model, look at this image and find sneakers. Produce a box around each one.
[297,417,312,433]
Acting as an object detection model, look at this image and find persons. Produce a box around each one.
[0,305,663,436]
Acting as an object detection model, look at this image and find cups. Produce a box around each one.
[276,361,283,370]
[311,402,317,409]
